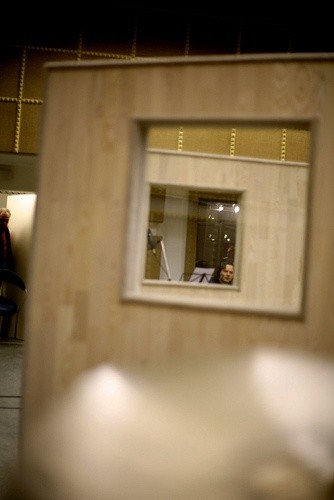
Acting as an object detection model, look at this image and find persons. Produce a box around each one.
[223,246,234,265]
[0,208,27,341]
[207,260,233,285]
